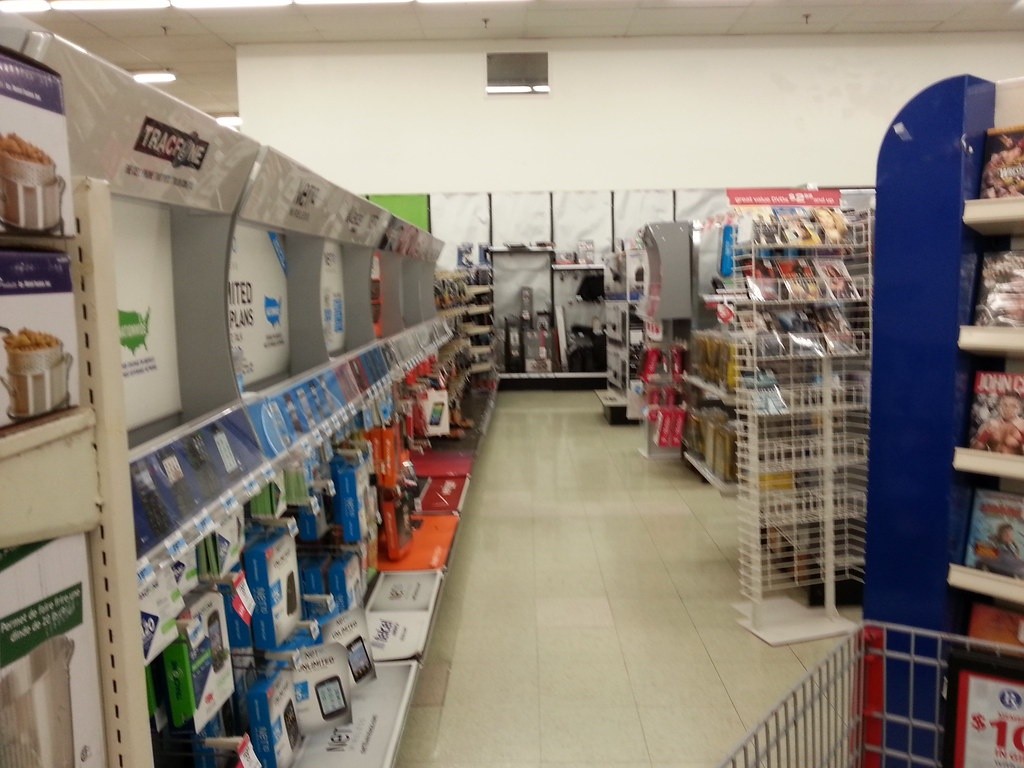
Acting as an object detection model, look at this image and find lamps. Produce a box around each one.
[135,68,178,83]
[485,52,549,93]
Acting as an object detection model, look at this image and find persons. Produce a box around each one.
[974,388,1024,455]
[979,520,1024,577]
[825,261,853,298]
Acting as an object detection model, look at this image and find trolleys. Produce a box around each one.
[712,621,1024,768]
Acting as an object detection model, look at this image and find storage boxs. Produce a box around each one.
[976,123,1024,197]
[970,251,1024,328]
[964,483,1024,581]
[966,368,1024,456]
[0,50,382,768]
[639,339,687,448]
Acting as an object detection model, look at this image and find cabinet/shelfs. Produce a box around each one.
[487,245,605,270]
[435,270,499,445]
[681,293,751,495]
[948,196,1024,610]
